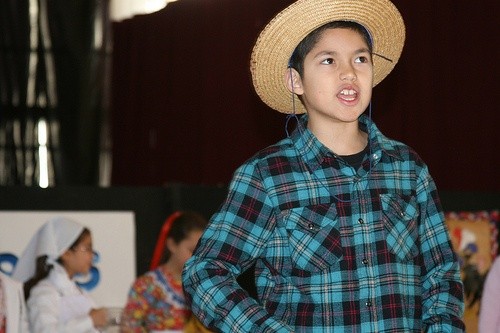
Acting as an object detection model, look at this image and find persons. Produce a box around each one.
[22,217,111,333]
[120,212,212,333]
[182,1,467,333]
[0,269,30,333]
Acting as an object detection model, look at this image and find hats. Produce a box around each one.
[250,0,405,117]
